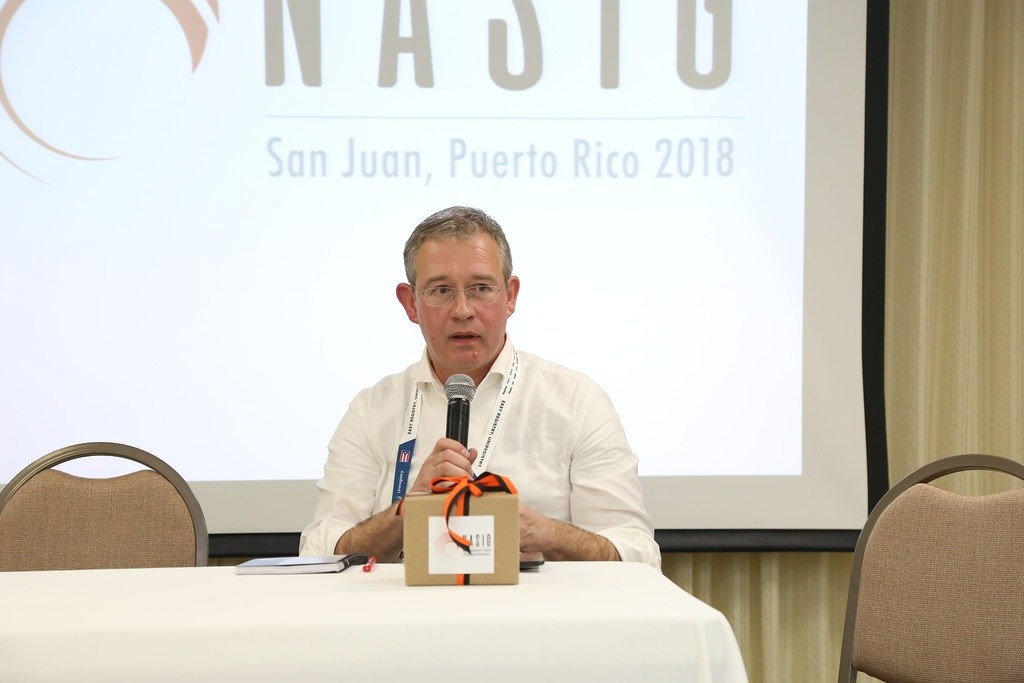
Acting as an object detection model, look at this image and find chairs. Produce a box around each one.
[837,454,1024,683]
[0,442,210,572]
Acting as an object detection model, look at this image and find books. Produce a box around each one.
[515,551,545,570]
[232,553,367,574]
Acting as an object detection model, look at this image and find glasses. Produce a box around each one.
[409,281,510,308]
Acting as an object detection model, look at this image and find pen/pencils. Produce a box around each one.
[362,556,376,572]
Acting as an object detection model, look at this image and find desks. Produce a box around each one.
[0,561,749,683]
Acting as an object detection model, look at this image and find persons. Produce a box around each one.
[299,206,662,574]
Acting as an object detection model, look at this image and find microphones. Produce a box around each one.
[444,374,476,451]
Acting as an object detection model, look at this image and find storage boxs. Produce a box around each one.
[404,491,521,586]
